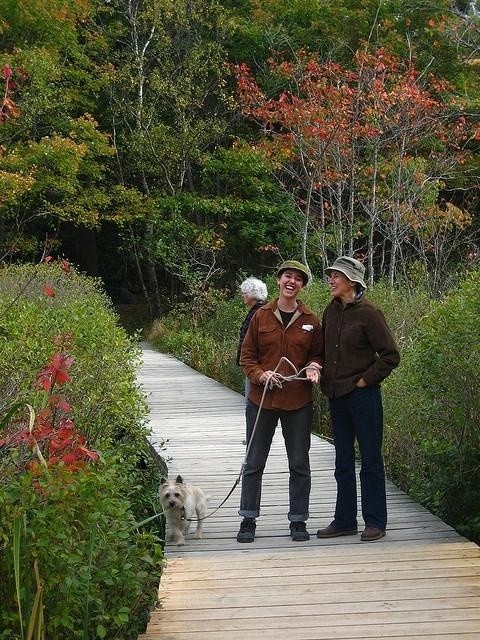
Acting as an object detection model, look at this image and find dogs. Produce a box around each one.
[158,474,212,546]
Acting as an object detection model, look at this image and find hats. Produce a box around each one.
[277,260,309,288]
[324,256,367,292]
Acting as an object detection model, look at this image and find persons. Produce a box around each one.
[236,260,323,542]
[236,274,268,409]
[316,255,401,543]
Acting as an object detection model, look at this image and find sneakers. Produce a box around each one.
[317,520,357,538]
[289,521,309,541]
[361,526,386,540]
[237,519,256,543]
